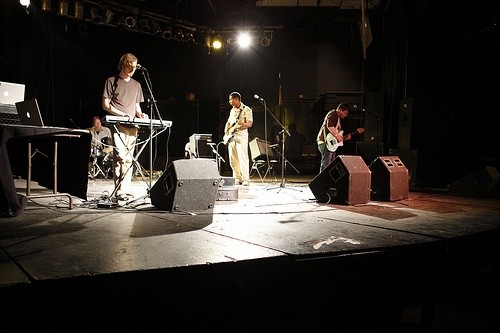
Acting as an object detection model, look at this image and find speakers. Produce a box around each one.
[193,134,213,157]
[150,158,220,211]
[308,140,409,204]
[249,138,275,161]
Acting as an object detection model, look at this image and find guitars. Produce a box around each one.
[325,127,365,152]
[222,105,246,146]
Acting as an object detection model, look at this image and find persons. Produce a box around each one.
[317,103,365,173]
[88,116,111,179]
[102,54,149,200]
[224,92,252,186]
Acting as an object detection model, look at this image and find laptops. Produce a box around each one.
[16,99,44,127]
[0,82,25,105]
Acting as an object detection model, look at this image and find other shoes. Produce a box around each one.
[242,181,250,186]
[118,193,126,199]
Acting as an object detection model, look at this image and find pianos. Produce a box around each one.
[100,114,173,196]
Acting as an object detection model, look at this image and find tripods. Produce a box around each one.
[259,99,304,194]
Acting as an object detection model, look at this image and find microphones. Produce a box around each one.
[269,143,279,148]
[137,64,146,70]
[254,94,265,102]
